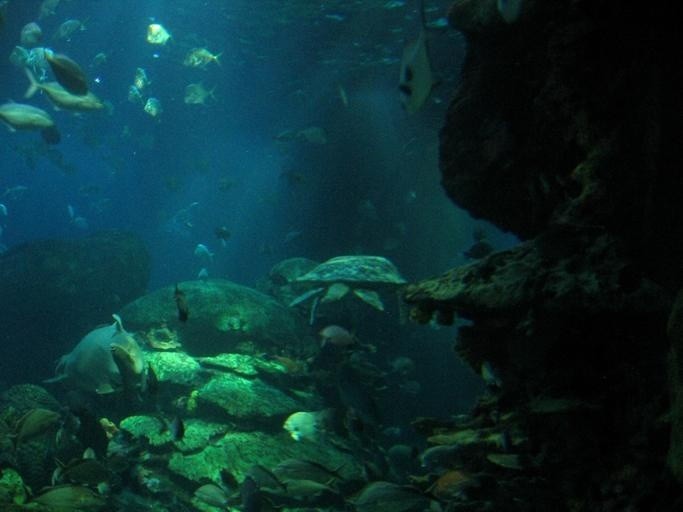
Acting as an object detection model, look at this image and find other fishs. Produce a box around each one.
[0,0,467,228]
[9,317,545,512]
